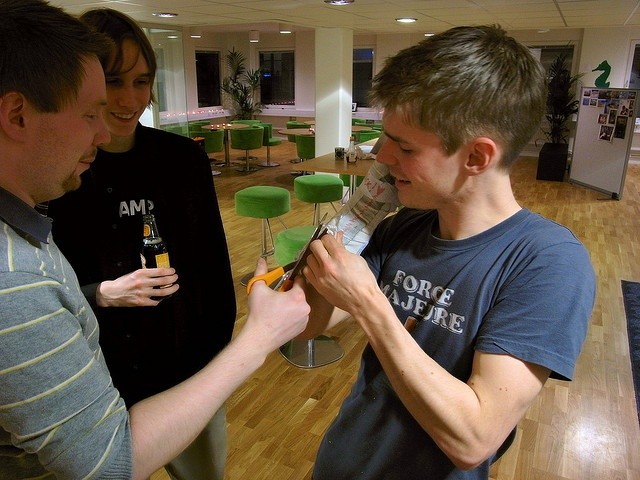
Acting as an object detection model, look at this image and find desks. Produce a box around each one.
[276,128,315,163]
[350,125,374,145]
[199,122,250,170]
[290,136,384,224]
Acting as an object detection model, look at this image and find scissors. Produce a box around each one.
[247,212,328,293]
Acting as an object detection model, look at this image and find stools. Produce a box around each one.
[295,172,344,225]
[236,184,289,287]
[274,224,345,369]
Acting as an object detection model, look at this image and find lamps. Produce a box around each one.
[152,12,179,19]
[249,30,260,43]
[186,26,206,42]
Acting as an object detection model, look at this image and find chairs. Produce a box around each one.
[229,126,264,173]
[230,120,263,160]
[354,122,376,145]
[285,120,311,163]
[168,124,187,137]
[352,118,366,125]
[189,121,210,131]
[189,121,218,165]
[252,123,283,169]
[292,133,315,176]
[372,124,382,130]
[351,130,381,143]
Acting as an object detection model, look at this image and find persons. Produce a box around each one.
[47,7,237,480]
[1,0,311,480]
[293,23,597,479]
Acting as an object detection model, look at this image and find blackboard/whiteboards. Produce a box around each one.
[567,86,640,199]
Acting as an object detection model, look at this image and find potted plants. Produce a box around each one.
[535,39,586,183]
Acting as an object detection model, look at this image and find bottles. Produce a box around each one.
[140,214,174,299]
[346,136,357,164]
